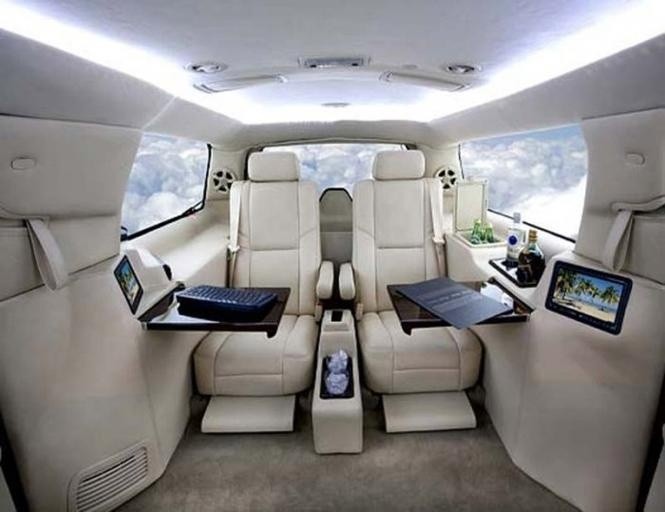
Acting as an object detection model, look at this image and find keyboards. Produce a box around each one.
[175,283,279,312]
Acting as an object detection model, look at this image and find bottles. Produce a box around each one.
[483,222,494,244]
[506,213,527,268]
[472,219,483,244]
[516,228,546,284]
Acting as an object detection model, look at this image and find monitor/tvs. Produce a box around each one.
[113,256,143,315]
[545,261,632,335]
